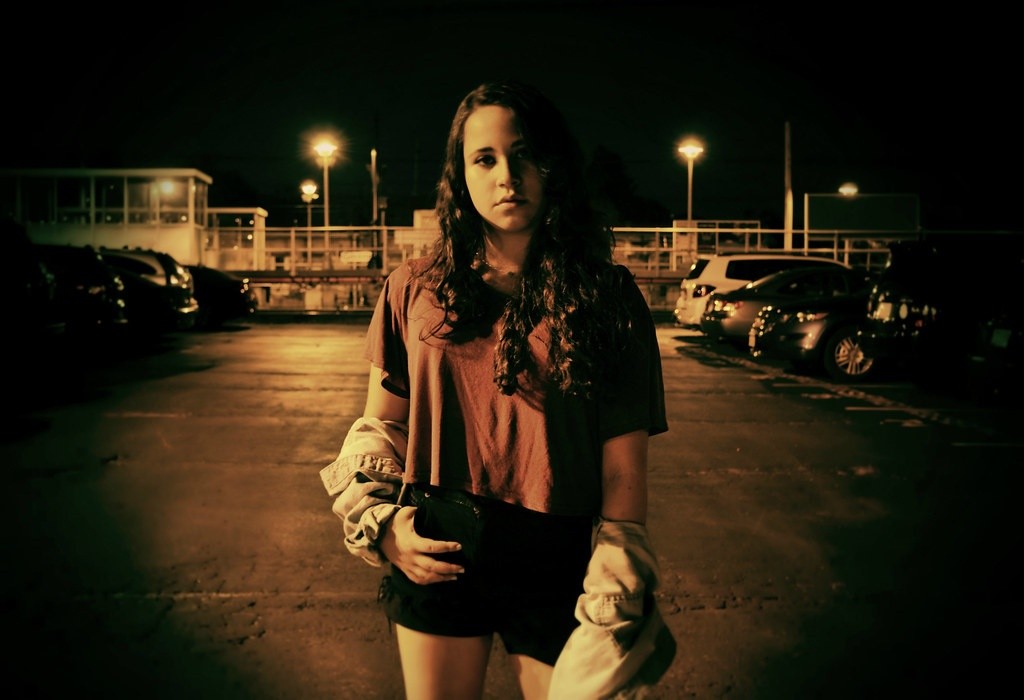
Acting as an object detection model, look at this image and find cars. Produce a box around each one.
[671,254,852,327]
[1,216,259,399]
[748,259,1024,385]
[699,265,900,365]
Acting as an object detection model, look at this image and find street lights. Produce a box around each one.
[677,139,707,251]
[314,140,339,269]
[300,180,318,269]
[839,182,859,247]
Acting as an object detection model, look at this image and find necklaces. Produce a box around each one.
[475,250,517,279]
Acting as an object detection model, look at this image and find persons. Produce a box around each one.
[330,81,670,700]
